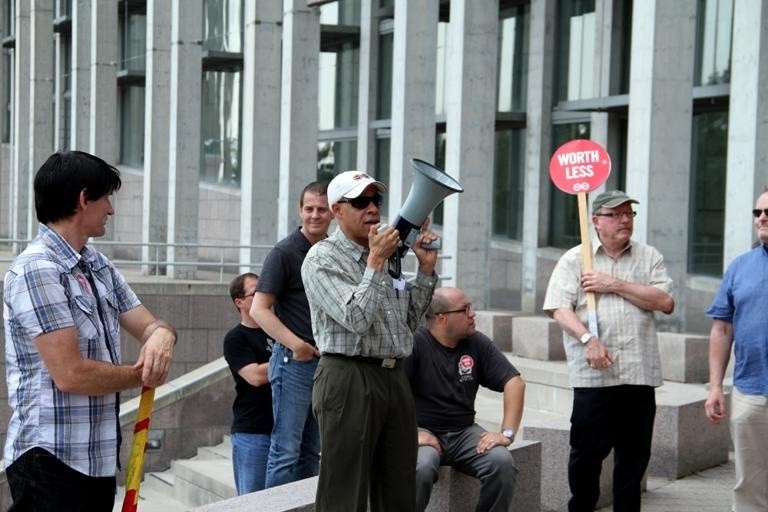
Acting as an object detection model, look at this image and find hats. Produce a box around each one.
[591,190,640,214]
[327,170,387,213]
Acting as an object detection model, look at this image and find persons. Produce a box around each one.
[1,148,176,512]
[703,185,767,511]
[220,272,279,498]
[398,286,528,512]
[297,169,442,512]
[541,189,676,512]
[246,178,335,490]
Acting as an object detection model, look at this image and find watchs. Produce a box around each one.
[499,428,515,442]
[578,331,593,346]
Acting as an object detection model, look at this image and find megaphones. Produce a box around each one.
[377,158,465,259]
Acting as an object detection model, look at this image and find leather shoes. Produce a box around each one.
[322,352,404,369]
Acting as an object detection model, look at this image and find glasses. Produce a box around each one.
[336,195,384,207]
[596,209,637,218]
[434,301,472,316]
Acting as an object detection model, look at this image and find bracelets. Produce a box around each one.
[141,319,178,347]
